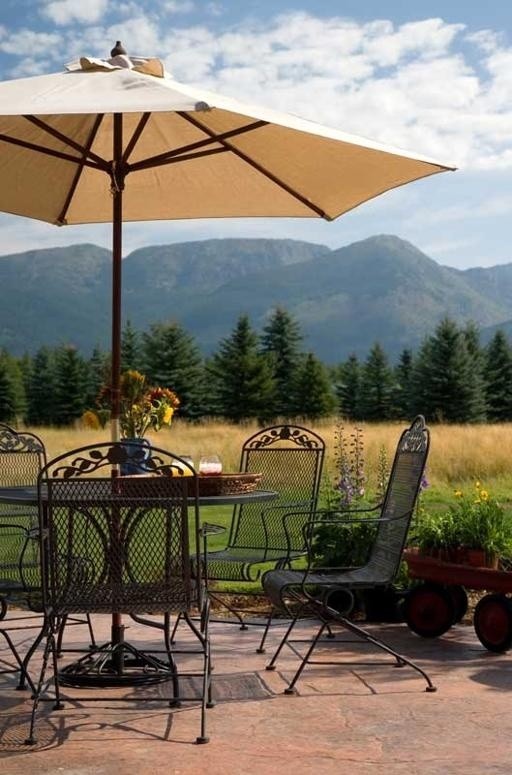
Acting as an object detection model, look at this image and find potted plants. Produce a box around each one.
[407,505,458,563]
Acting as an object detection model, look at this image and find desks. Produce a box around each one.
[1,482,279,689]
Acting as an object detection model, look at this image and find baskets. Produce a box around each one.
[119,473,261,495]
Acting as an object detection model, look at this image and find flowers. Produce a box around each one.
[448,479,512,571]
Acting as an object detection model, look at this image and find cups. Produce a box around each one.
[171,454,195,477]
[198,454,222,475]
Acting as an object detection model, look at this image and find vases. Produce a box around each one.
[464,547,499,569]
[116,438,151,477]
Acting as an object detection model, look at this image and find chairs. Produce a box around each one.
[1,422,99,700]
[260,413,436,697]
[171,423,324,656]
[23,440,214,746]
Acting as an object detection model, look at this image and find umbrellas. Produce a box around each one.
[0,40,462,627]
